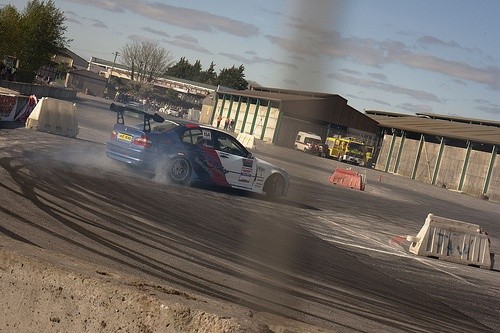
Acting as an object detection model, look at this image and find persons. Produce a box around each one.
[102,86,187,120]
[216,116,236,131]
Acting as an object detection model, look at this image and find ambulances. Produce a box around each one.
[294,131,324,157]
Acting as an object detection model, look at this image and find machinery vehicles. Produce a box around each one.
[324,134,374,167]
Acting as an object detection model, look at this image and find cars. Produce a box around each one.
[106,103,290,203]
[159,108,179,117]
